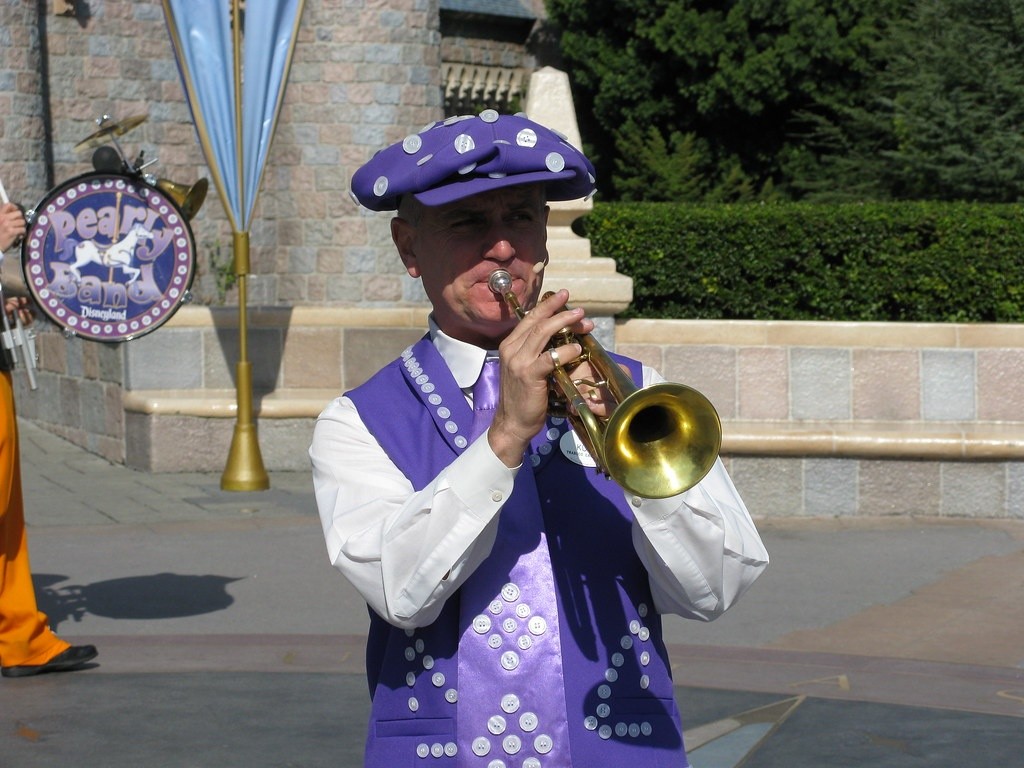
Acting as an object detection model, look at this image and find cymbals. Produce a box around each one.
[74,111,151,153]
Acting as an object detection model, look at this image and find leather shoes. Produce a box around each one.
[1,644,99,677]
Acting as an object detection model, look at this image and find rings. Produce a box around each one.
[549,348,561,368]
[588,390,598,402]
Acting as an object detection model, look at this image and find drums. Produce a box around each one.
[21,171,198,346]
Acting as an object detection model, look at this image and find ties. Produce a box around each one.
[456,358,574,768]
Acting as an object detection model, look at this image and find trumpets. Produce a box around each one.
[488,270,723,501]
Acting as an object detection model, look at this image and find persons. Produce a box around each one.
[0,201,98,679]
[309,109,770,768]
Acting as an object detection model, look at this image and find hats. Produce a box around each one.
[348,109,598,212]
